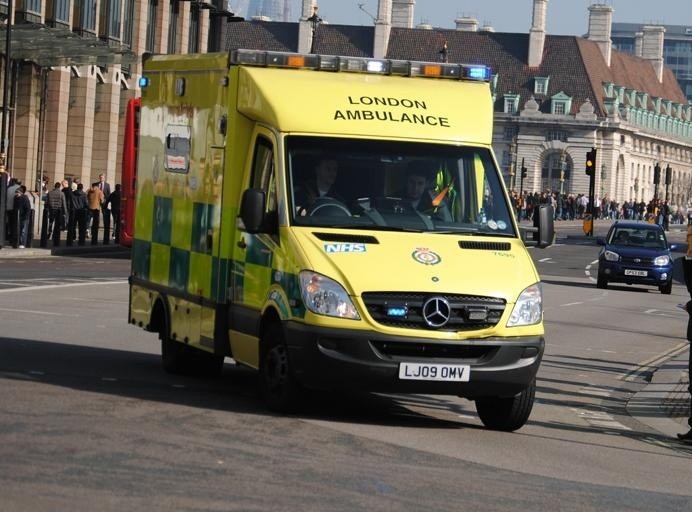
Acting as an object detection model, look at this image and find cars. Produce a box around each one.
[597,221,678,294]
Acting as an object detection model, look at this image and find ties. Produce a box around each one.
[100,183,103,191]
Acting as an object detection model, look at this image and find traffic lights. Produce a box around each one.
[521,167,527,178]
[586,152,593,175]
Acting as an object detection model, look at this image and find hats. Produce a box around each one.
[14,187,24,195]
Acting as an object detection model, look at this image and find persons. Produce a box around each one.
[296,152,349,216]
[381,159,455,220]
[677,224,692,440]
[640,232,657,242]
[1,152,122,250]
[484,185,691,232]
[614,230,634,243]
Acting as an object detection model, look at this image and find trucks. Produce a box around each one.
[128,48,545,430]
